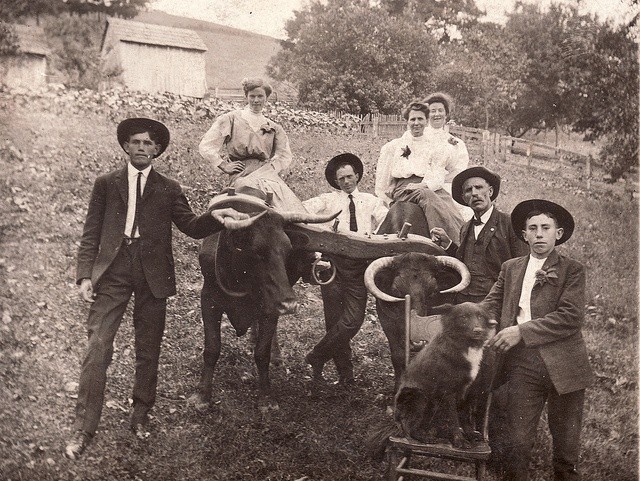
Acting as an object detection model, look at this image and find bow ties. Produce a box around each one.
[470,217,482,225]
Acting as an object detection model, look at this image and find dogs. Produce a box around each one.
[368,300,498,465]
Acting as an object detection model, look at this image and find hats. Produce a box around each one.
[452,166,500,205]
[325,154,363,189]
[511,199,574,245]
[117,118,169,158]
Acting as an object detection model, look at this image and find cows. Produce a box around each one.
[188,207,342,413]
[364,201,471,403]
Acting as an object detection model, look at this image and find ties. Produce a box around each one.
[349,194,358,232]
[130,172,142,238]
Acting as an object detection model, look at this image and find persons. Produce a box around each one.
[199,77,321,286]
[481,199,589,481]
[64,117,240,461]
[402,92,475,222]
[301,154,390,388]
[428,167,528,436]
[376,102,466,251]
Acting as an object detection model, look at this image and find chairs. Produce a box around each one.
[389,295,493,481]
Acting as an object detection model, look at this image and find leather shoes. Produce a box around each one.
[62,430,91,460]
[304,354,325,381]
[132,422,150,443]
